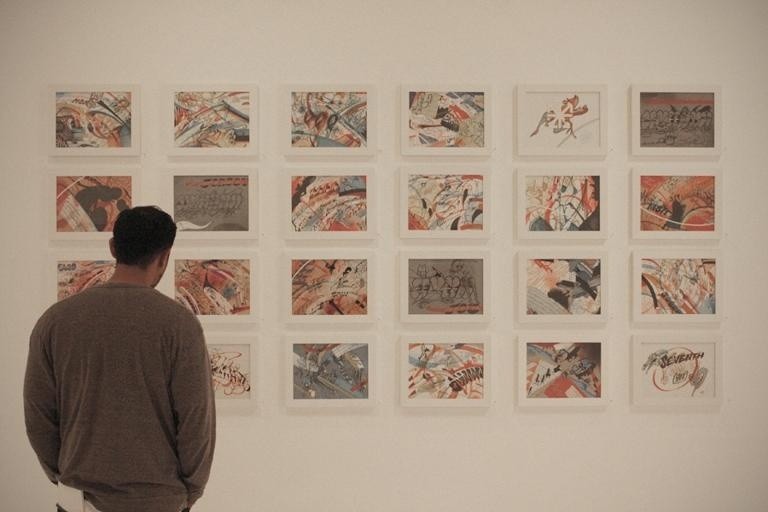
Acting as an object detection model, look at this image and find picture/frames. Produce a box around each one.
[279,80,376,159]
[518,250,607,324]
[46,167,140,244]
[631,249,720,326]
[46,82,142,158]
[397,171,492,240]
[512,84,610,163]
[401,335,494,409]
[162,169,261,240]
[284,335,379,409]
[631,333,720,407]
[632,167,719,242]
[164,250,262,325]
[202,332,262,408]
[517,171,608,241]
[400,88,491,160]
[162,81,261,159]
[517,334,609,405]
[628,84,720,158]
[280,167,375,242]
[284,250,376,325]
[47,250,118,312]
[397,253,489,323]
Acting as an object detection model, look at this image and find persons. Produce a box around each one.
[24,205,216,512]
